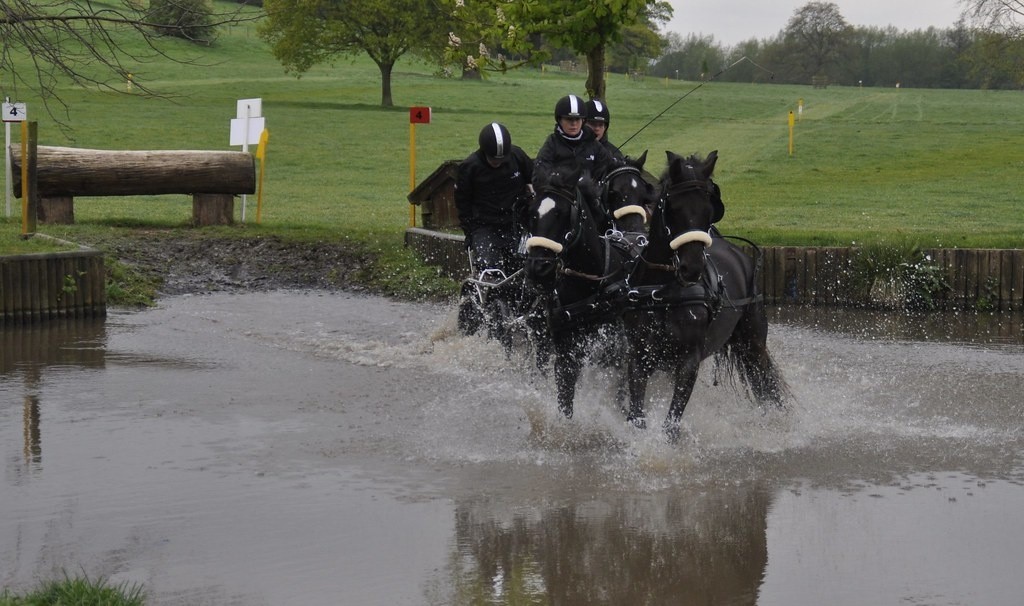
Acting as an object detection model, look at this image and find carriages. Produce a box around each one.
[457,149,806,444]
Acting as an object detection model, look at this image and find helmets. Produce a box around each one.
[479,123,511,159]
[585,101,610,133]
[554,94,586,122]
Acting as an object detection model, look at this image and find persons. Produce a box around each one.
[532,95,623,184]
[455,123,533,274]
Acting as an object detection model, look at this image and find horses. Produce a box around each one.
[524,150,793,445]
